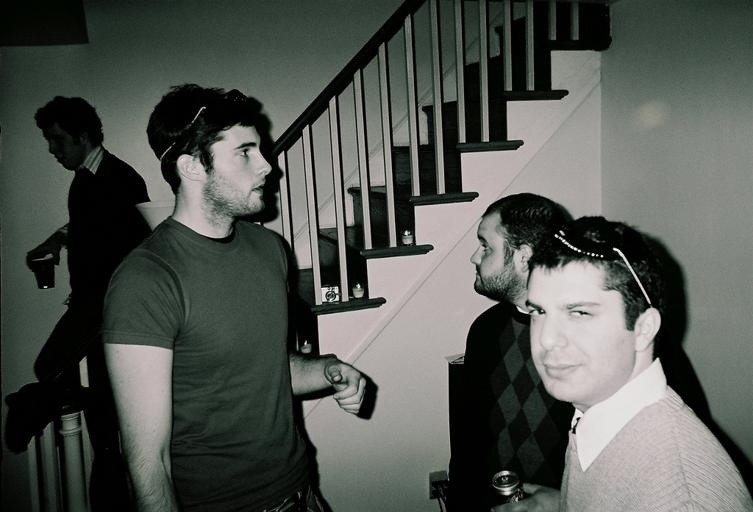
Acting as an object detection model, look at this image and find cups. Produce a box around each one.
[31,257,56,289]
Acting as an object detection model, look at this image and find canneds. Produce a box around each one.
[490,470,524,504]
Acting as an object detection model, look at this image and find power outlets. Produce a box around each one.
[430,471,449,499]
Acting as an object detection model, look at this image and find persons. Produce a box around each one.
[95,80,369,510]
[442,192,576,511]
[486,213,753,511]
[24,93,164,511]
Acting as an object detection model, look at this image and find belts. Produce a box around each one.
[262,490,304,512]
